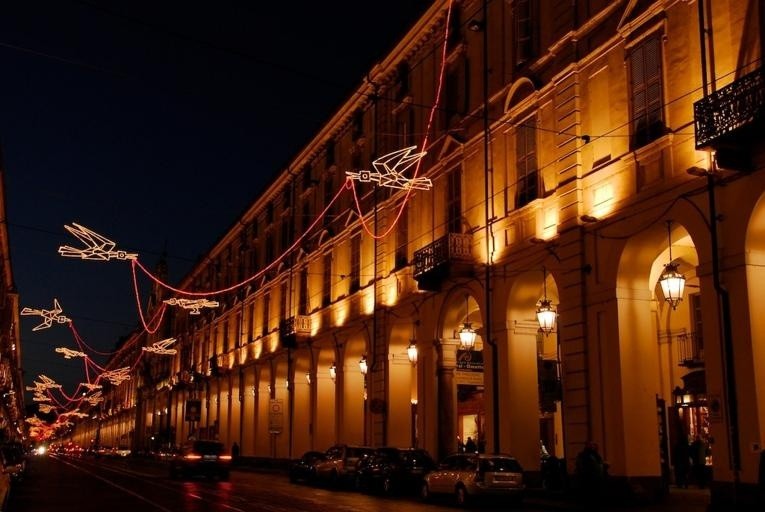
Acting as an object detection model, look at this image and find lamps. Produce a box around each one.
[407,318,420,367]
[660,219,686,311]
[458,294,477,352]
[536,266,558,336]
[359,353,368,374]
[329,360,337,380]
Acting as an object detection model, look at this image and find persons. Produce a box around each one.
[455,436,466,454]
[463,437,477,453]
[662,434,708,489]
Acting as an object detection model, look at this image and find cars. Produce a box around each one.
[40,442,233,477]
[291,439,532,507]
